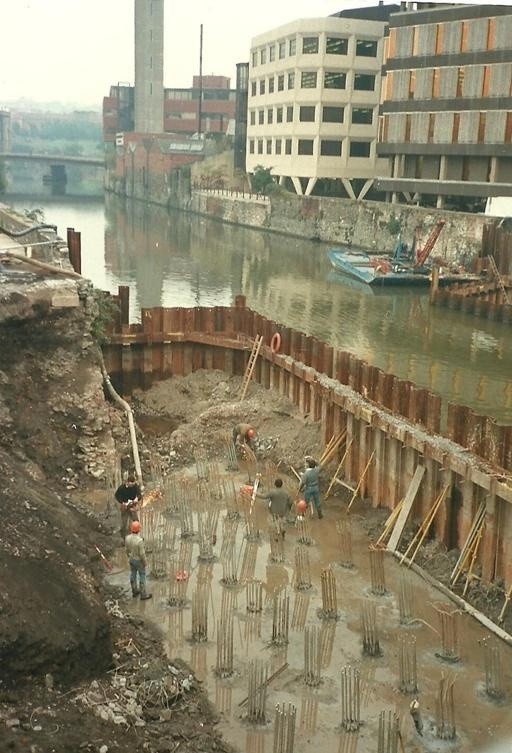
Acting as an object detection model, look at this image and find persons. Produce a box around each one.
[231,421,256,461]
[124,519,154,601]
[251,477,294,543]
[114,475,146,546]
[296,453,328,520]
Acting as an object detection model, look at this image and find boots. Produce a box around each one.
[317,509,323,519]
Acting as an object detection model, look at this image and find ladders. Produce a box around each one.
[237,334,263,400]
[488,255,510,304]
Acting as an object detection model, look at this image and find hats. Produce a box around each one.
[127,476,135,482]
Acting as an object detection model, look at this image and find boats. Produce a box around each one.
[324,220,483,287]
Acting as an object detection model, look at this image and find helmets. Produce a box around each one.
[247,429,255,440]
[130,521,141,533]
